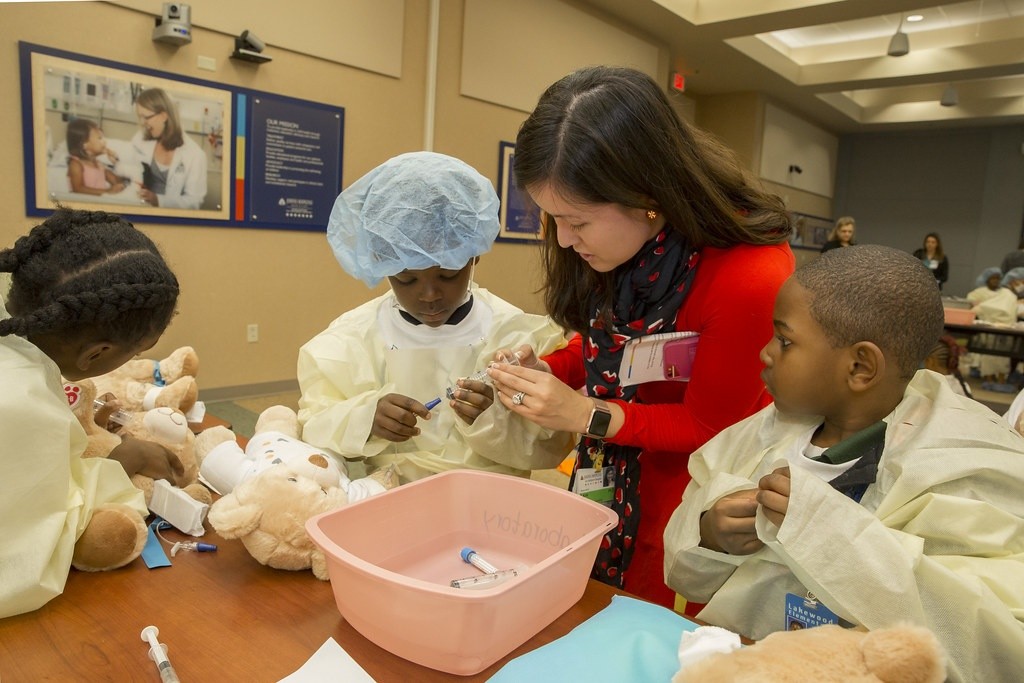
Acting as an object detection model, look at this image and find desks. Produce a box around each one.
[0,415,753,682]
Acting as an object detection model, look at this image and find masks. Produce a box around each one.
[1011,285,1024,298]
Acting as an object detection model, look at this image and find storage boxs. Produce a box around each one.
[944,307,979,324]
[305,468,619,678]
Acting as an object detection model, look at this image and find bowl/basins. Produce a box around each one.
[305,468,620,676]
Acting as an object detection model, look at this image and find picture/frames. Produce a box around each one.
[495,139,548,246]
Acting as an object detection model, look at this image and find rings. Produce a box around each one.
[512,392,525,405]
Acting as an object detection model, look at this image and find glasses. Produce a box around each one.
[138,111,161,121]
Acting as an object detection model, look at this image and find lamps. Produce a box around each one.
[887,14,908,57]
[939,84,960,107]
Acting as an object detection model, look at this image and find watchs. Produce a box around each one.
[581,397,611,439]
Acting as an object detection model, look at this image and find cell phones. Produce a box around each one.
[662,336,700,379]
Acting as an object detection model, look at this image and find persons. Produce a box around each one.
[66,119,126,196]
[296,150,576,480]
[821,217,856,253]
[661,244,1024,683]
[105,88,208,209]
[0,206,184,618]
[913,233,948,289]
[486,68,799,618]
[924,335,971,399]
[603,468,614,486]
[790,621,803,630]
[965,239,1024,393]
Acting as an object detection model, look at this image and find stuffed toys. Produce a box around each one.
[193,404,399,581]
[61,346,213,573]
[675,619,946,683]
